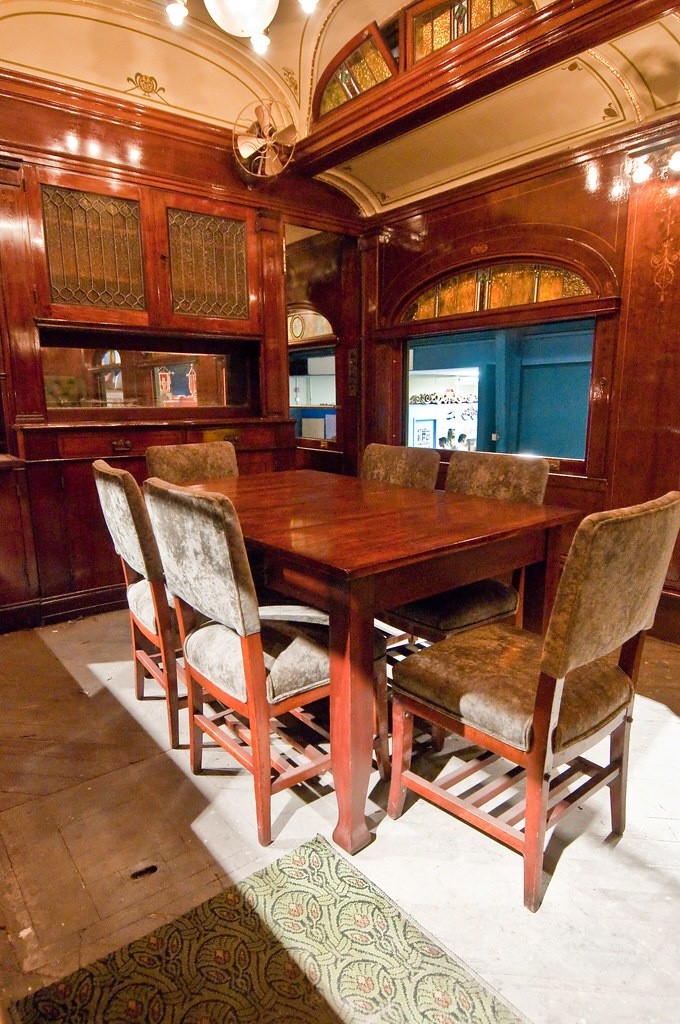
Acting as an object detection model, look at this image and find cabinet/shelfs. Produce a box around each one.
[2,164,290,624]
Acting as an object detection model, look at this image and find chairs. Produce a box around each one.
[142,476,387,851]
[142,423,553,645]
[90,459,282,753]
[384,488,680,913]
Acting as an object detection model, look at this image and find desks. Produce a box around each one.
[168,465,583,857]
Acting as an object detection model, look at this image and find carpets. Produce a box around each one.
[8,832,536,1024]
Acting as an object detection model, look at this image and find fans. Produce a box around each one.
[232,95,299,180]
